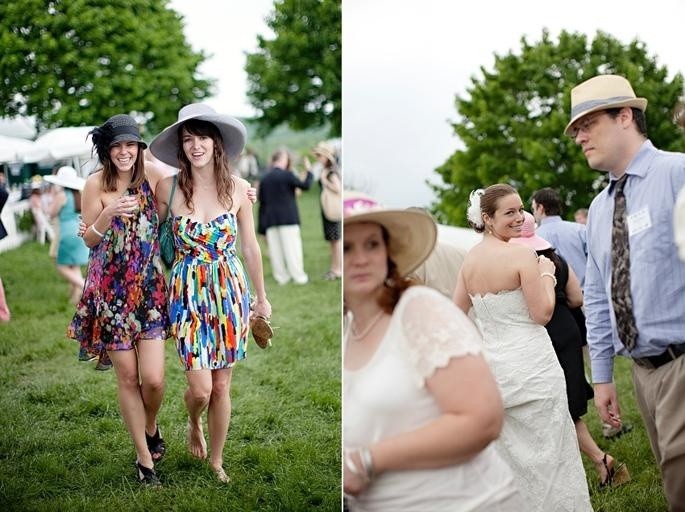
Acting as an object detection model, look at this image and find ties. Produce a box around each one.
[612,174,638,353]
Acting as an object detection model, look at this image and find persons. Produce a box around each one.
[574,208,588,225]
[511,210,632,494]
[29,179,58,256]
[452,185,594,512]
[343,191,525,512]
[257,150,313,286]
[40,170,89,305]
[532,190,634,439]
[314,139,342,283]
[564,75,683,512]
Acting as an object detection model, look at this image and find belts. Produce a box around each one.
[632,344,685,370]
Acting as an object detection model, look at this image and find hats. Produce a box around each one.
[343,190,437,277]
[150,103,247,170]
[86,114,148,164]
[313,143,337,167]
[508,210,552,251]
[563,74,647,135]
[43,166,86,191]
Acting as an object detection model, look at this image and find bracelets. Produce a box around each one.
[358,447,375,480]
[541,272,557,287]
[92,225,104,238]
[344,452,373,483]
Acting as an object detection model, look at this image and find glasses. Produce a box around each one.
[570,111,606,138]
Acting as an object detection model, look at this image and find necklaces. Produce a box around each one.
[348,308,384,341]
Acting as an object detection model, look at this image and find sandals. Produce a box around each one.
[136,459,162,492]
[146,424,165,461]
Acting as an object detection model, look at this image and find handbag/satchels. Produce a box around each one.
[320,170,342,222]
[158,174,177,267]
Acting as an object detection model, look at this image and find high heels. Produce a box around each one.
[251,319,274,349]
[599,454,630,491]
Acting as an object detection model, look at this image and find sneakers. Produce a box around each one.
[602,421,633,441]
